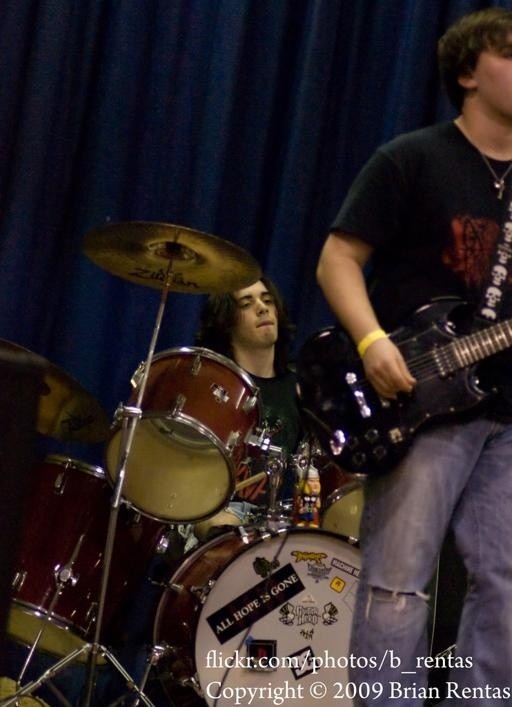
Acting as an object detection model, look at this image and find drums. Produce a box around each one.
[101,346,268,526]
[318,481,365,542]
[153,519,362,707]
[0,452,171,664]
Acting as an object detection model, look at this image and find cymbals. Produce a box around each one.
[0,338,112,444]
[77,221,263,294]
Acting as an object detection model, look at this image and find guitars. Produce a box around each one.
[295,296,512,480]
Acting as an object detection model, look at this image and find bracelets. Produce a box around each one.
[357,329,387,358]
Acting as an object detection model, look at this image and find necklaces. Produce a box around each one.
[475,138,512,200]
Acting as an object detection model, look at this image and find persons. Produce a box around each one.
[315,6,512,707]
[193,273,300,541]
